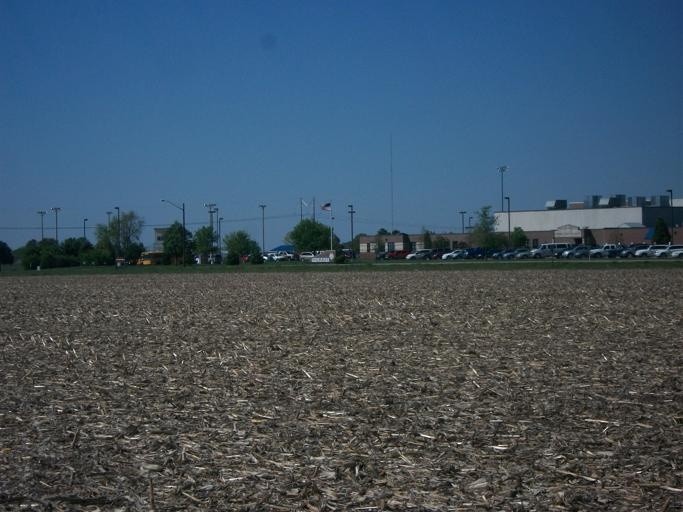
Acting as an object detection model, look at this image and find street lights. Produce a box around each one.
[496,164,509,214]
[504,195,511,246]
[664,188,673,246]
[159,198,186,268]
[457,210,467,233]
[347,203,355,255]
[35,204,125,256]
[213,205,223,258]
[468,216,474,234]
[258,203,266,254]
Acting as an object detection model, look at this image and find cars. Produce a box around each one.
[192,248,356,264]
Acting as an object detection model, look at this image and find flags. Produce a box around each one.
[320,202,331,211]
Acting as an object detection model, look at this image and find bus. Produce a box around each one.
[127,251,166,266]
